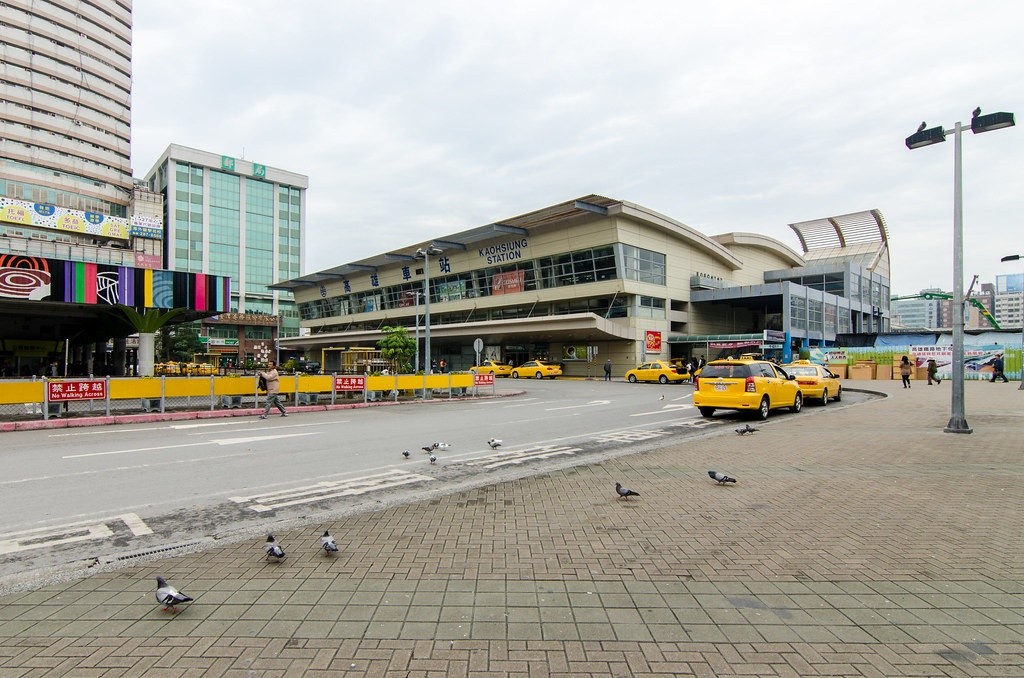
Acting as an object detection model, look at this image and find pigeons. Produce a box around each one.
[321,530,339,557]
[658,395,664,400]
[614,479,641,499]
[973,107,981,117]
[435,442,452,449]
[734,428,748,436]
[153,575,193,615]
[402,449,411,458]
[265,530,286,566]
[417,244,434,253]
[708,470,738,486]
[744,424,760,435]
[429,452,436,465]
[917,121,927,132]
[422,444,439,453]
[488,437,503,449]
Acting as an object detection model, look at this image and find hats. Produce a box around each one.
[927,359,935,362]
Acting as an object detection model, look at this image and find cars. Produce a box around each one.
[509,359,563,380]
[290,356,322,375]
[670,357,689,367]
[693,354,803,421]
[625,359,691,384]
[777,359,843,406]
[469,359,513,378]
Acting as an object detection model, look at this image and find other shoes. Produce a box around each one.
[989,380,995,382]
[261,415,267,418]
[281,411,286,416]
[938,380,941,384]
[904,386,907,388]
[909,385,911,388]
[928,383,932,385]
[1002,380,1009,383]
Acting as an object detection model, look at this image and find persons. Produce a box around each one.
[440,358,446,373]
[990,354,1008,383]
[604,359,611,381]
[432,358,438,372]
[767,357,782,364]
[509,358,513,366]
[683,355,706,383]
[926,357,941,385]
[900,357,912,388]
[473,357,488,365]
[258,361,288,419]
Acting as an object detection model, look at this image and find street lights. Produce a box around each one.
[904,111,1018,434]
[406,292,426,375]
[413,248,444,400]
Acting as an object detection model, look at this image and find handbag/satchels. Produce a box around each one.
[934,372,941,380]
[257,375,267,391]
